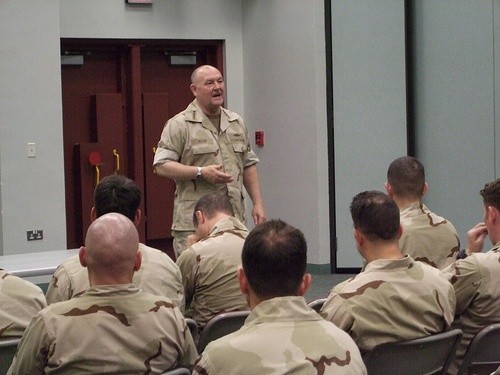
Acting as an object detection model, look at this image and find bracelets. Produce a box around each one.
[195,167,202,179]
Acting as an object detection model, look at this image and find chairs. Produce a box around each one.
[363,328,465,375]
[457,321,500,375]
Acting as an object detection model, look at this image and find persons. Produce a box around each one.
[442,177,500,375]
[193,220,367,375]
[152,64,265,257]
[385,157,461,273]
[176,192,250,326]
[46,174,186,315]
[0,271,46,338]
[8,213,199,375]
[320,189,455,353]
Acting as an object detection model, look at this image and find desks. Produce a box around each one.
[0,249,84,278]
